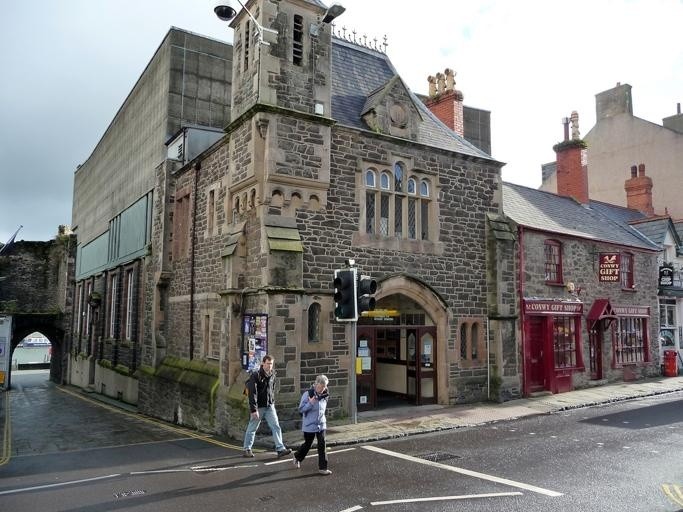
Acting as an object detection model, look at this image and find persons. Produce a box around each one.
[244,354,291,457]
[290,374,332,475]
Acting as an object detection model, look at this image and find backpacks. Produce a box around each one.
[242,371,261,397]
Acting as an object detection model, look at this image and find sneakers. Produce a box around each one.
[278,448,292,457]
[291,451,301,468]
[318,469,332,475]
[243,450,254,457]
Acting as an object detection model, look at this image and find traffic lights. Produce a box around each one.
[358,275,376,316]
[334,270,354,321]
